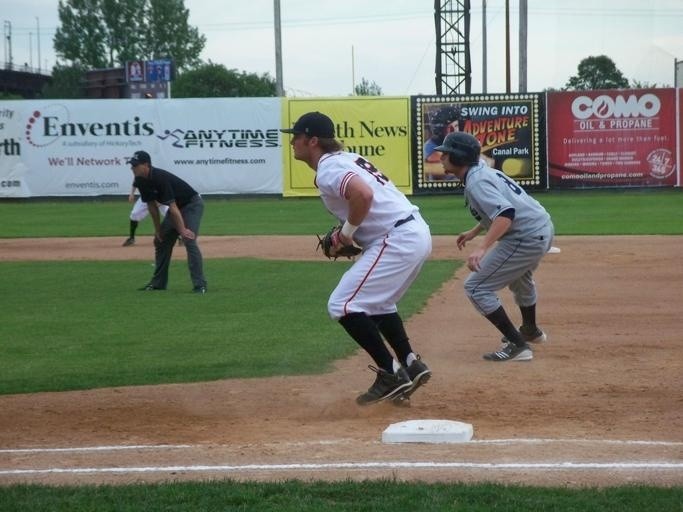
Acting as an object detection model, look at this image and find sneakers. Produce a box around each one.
[138,285,153,290]
[192,286,207,295]
[391,354,432,401]
[356,365,413,406]
[123,238,134,246]
[483,341,534,361]
[501,326,547,344]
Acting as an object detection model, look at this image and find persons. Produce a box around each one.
[122,179,185,248]
[422,106,494,182]
[124,150,209,293]
[436,126,555,361]
[281,112,434,407]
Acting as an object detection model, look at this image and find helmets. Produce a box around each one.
[433,132,480,163]
[429,107,465,141]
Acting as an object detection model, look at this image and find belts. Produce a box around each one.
[395,215,414,227]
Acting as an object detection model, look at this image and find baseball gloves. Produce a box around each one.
[316,226,361,261]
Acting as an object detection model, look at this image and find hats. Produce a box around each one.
[126,151,150,167]
[280,112,335,138]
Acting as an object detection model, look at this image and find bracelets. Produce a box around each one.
[342,217,359,240]
